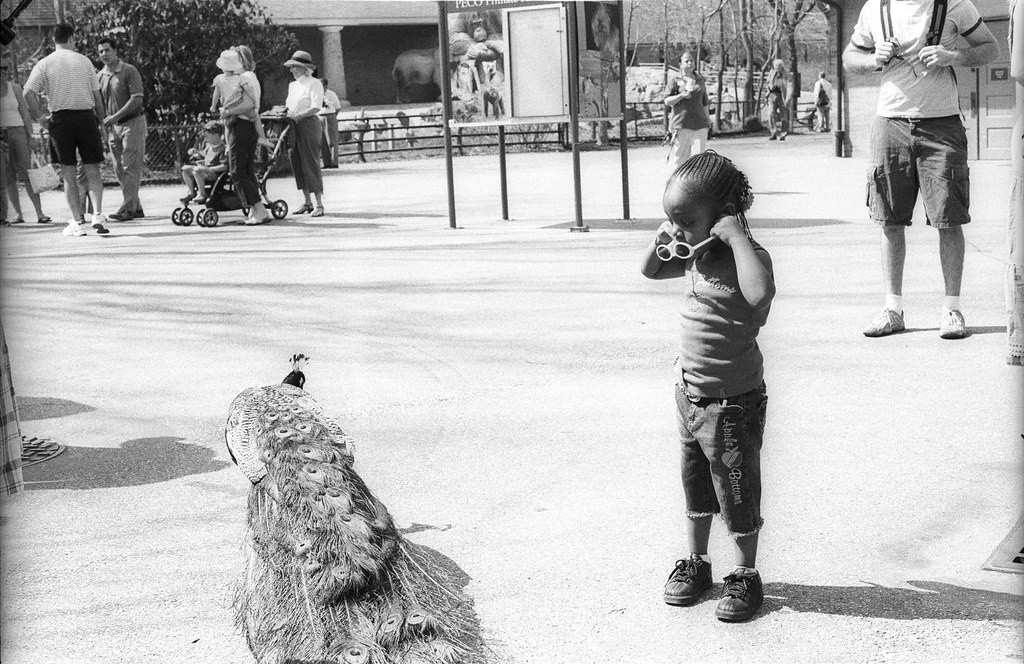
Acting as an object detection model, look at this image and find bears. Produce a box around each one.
[479,58,505,85]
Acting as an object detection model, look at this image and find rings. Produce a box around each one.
[927,56,933,62]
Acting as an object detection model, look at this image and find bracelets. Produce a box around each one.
[226,109,229,116]
[35,114,45,121]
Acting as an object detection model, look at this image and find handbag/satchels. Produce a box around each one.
[814,81,829,107]
[27,149,61,193]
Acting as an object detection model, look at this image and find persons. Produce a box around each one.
[814,72,833,132]
[318,79,342,169]
[640,148,776,620]
[0,23,148,236]
[708,86,736,130]
[843,0,1001,337]
[210,46,274,225]
[180,120,229,201]
[765,59,794,141]
[663,51,712,168]
[284,51,325,216]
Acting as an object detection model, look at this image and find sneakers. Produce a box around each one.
[714,566,765,621]
[862,307,905,336]
[662,554,714,606]
[938,308,967,339]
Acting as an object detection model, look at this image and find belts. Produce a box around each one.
[118,107,146,124]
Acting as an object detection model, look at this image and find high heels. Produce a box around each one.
[291,202,314,214]
[310,203,325,217]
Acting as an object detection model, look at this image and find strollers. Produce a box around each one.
[171,114,290,229]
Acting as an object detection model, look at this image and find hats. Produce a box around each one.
[230,44,256,70]
[283,51,317,70]
[216,49,242,72]
[196,120,224,136]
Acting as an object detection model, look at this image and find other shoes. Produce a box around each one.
[62,219,88,237]
[769,134,785,140]
[11,218,24,223]
[236,214,273,225]
[131,210,145,218]
[192,193,206,204]
[84,212,93,222]
[0,219,11,226]
[37,216,53,223]
[91,214,110,233]
[108,208,134,221]
[179,194,196,205]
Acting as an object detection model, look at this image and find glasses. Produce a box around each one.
[655,234,717,262]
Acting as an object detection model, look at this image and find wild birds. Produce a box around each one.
[224,354,484,664]
